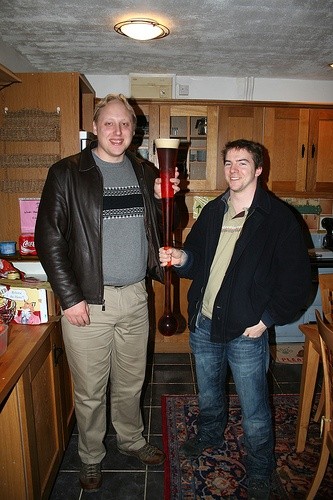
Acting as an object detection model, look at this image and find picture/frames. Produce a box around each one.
[129,72,177,98]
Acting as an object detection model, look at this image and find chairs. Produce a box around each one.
[292,272,333,500]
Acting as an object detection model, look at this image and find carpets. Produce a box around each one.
[159,391,323,500]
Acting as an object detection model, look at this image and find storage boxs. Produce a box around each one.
[0,278,58,324]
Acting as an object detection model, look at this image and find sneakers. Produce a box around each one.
[79,462,102,492]
[178,435,225,460]
[248,478,271,500]
[118,443,166,466]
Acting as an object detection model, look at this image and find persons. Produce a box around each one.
[35,94,180,490]
[159,138,320,500]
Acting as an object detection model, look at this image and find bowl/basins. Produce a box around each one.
[0,296,16,326]
[197,151,206,162]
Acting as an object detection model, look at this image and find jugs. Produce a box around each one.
[195,117,207,135]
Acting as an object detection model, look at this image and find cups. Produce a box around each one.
[172,128,178,136]
[0,241,17,255]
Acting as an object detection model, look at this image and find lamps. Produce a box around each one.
[114,18,171,41]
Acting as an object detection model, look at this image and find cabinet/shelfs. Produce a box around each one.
[261,106,333,198]
[19,324,77,500]
[214,104,261,191]
[1,70,97,250]
[128,102,215,193]
